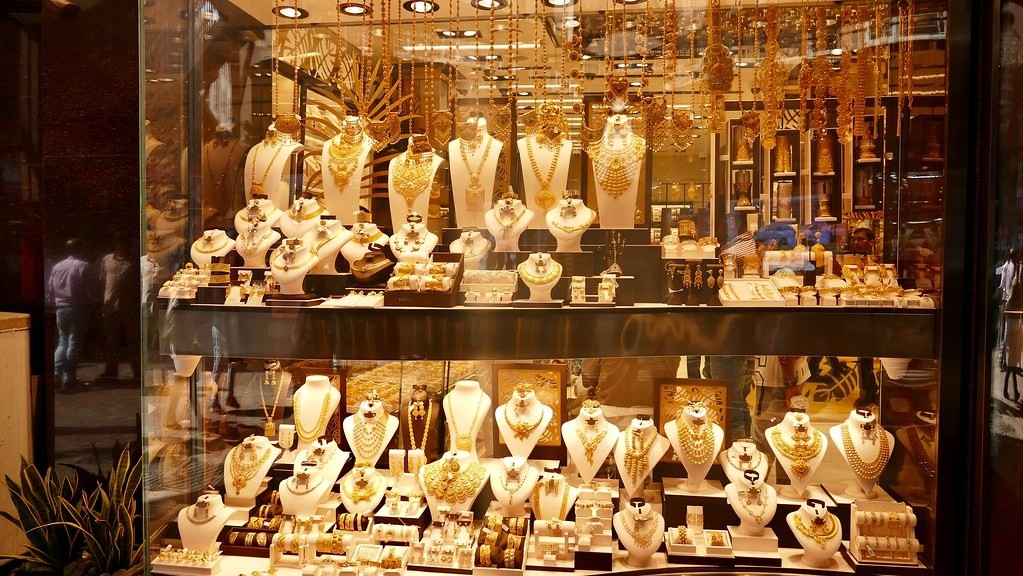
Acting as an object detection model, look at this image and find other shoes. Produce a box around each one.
[62,380,80,389]
[1015,393,1020,402]
[1004,391,1009,398]
[96,375,118,382]
[54,376,63,388]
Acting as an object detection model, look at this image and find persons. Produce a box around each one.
[799,237,848,381]
[46,237,94,392]
[752,222,811,486]
[710,232,754,445]
[140,237,246,432]
[852,228,879,406]
[993,247,1023,404]
[100,232,140,381]
[686,356,712,380]
[581,357,681,408]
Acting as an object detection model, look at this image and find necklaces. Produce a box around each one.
[187,0,914,548]
[164,209,189,221]
[146,238,177,252]
[206,141,238,194]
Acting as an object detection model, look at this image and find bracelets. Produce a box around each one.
[783,372,798,386]
[159,482,912,570]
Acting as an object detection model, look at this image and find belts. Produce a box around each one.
[56,305,85,308]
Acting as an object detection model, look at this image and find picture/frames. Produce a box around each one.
[577,94,654,228]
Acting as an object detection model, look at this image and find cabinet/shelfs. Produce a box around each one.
[136,0,982,576]
